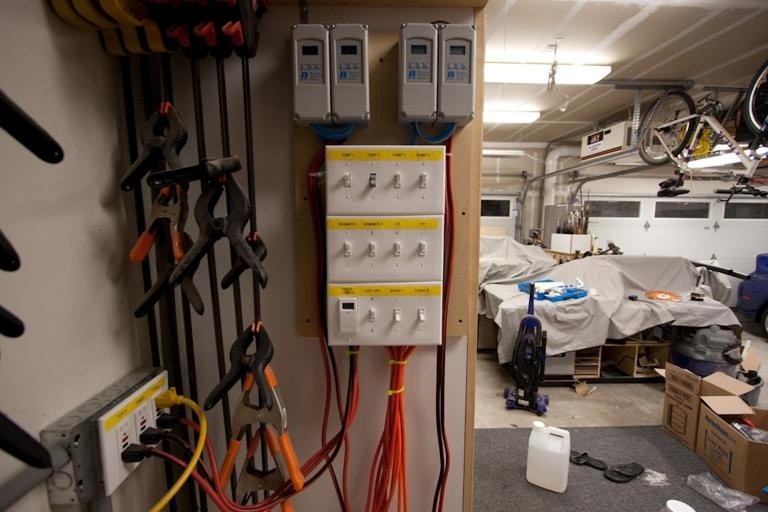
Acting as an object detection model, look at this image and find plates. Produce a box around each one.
[645,289,682,302]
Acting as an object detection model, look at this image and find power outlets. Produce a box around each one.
[98,370,172,498]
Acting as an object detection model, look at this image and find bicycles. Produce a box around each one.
[630,58,767,202]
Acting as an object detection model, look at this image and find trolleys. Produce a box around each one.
[503,282,551,416]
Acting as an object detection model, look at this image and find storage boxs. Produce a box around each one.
[580,120,665,164]
[551,232,599,255]
[654,360,768,504]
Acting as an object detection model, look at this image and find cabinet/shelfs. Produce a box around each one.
[527,295,672,385]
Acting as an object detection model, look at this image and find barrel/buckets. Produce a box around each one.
[525,421,571,494]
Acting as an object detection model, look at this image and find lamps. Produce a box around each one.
[484,61,612,85]
[483,110,541,124]
